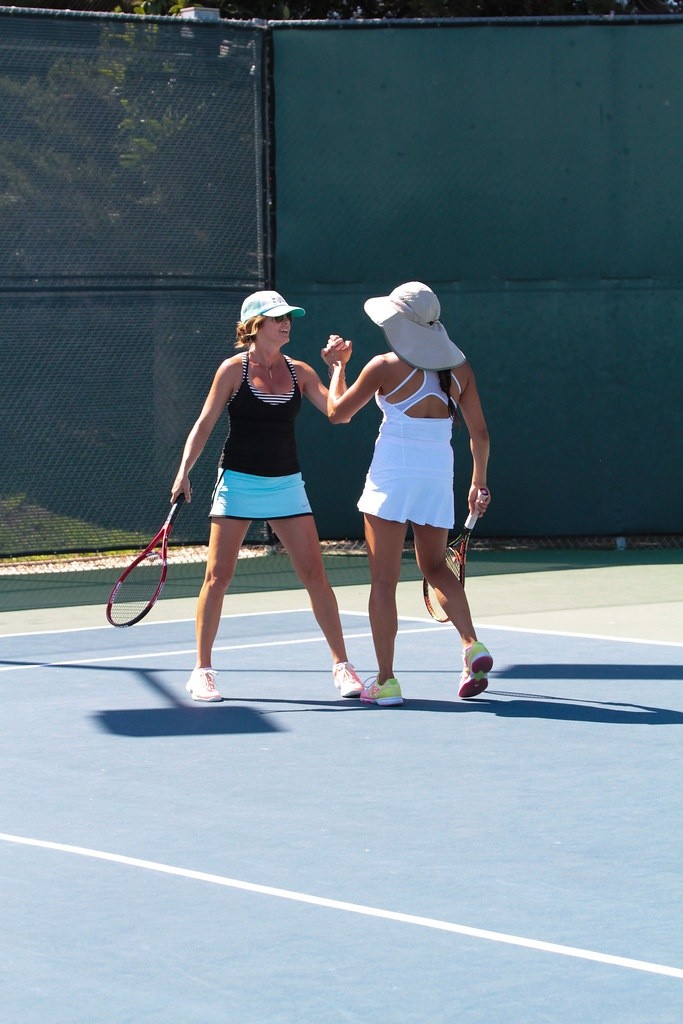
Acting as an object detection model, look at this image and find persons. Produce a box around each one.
[170,282,493,705]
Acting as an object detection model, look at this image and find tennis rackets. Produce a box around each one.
[421,486,491,625]
[103,483,191,629]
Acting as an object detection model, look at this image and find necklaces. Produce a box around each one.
[257,360,276,378]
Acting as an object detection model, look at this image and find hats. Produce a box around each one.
[241,290,305,323]
[364,281,466,371]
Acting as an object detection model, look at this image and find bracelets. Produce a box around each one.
[328,370,346,381]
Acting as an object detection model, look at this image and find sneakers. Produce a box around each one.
[458,642,493,697]
[360,674,403,705]
[332,662,364,696]
[186,667,221,701]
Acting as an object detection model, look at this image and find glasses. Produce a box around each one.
[267,313,292,323]
